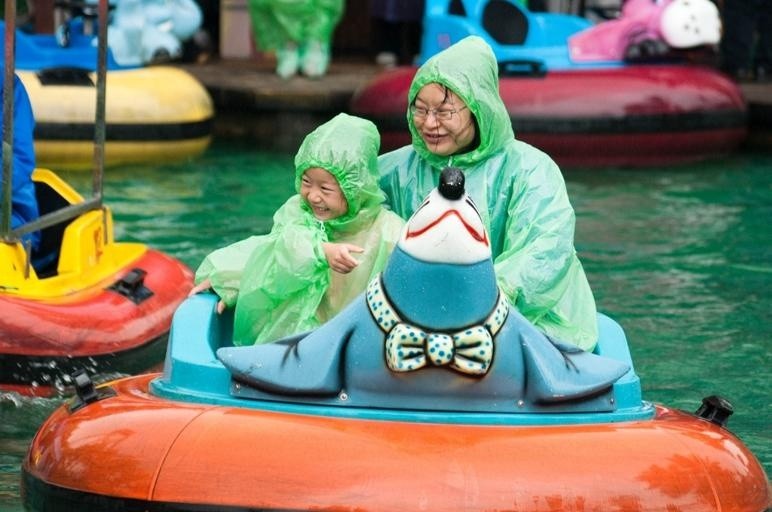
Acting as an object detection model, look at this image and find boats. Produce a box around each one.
[23,168,772,512]
[347,0,750,167]
[1,16,215,170]
[0,0,198,398]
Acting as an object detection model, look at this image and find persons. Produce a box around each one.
[232,110,408,347]
[187,34,602,355]
[0,62,42,261]
[244,0,347,79]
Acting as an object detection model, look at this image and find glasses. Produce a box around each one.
[408,104,468,120]
